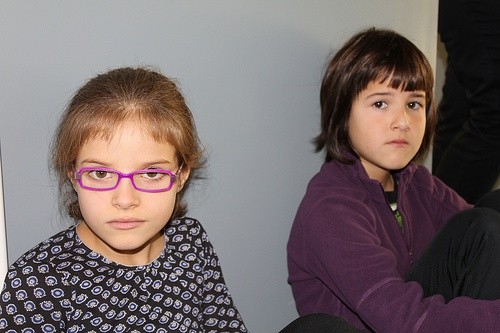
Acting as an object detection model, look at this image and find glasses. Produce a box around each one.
[72,160,185,192]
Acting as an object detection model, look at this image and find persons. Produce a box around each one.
[287,25,500,333]
[0,66,366,333]
[432,0,500,205]
